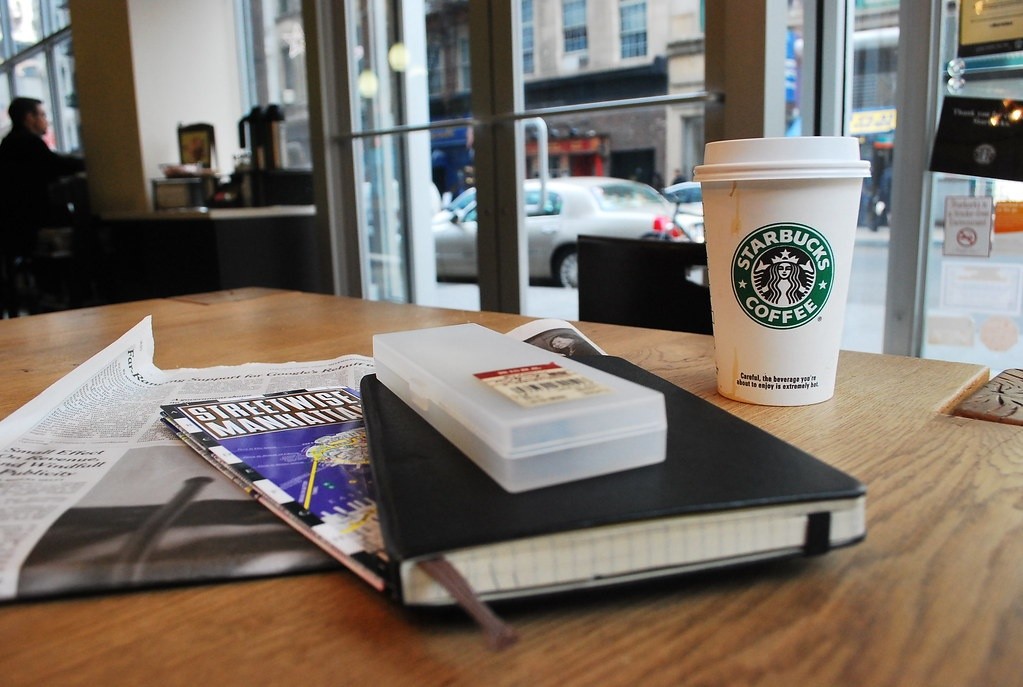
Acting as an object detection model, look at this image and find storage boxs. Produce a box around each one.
[372,324,668,493]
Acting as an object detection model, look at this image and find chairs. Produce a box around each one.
[576,233,714,336]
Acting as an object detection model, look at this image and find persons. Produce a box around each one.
[429,146,458,207]
[547,331,603,355]
[0,98,99,304]
[866,160,885,230]
[631,166,688,199]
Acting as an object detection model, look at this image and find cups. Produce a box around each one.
[691,136,875,407]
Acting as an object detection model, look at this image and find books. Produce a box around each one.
[151,356,867,623]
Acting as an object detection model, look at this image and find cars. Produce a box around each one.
[363,169,704,291]
[869,165,894,230]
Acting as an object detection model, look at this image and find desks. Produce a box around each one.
[95,205,318,294]
[1,286,1023,687]
